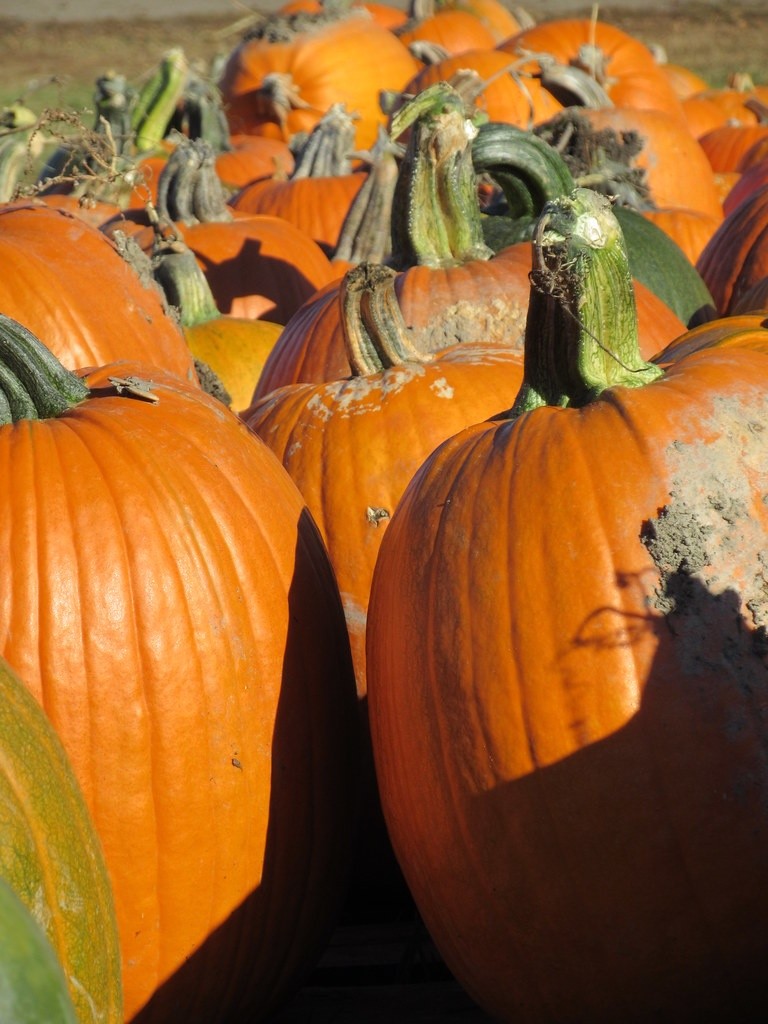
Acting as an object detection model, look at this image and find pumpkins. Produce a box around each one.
[0,2,766,1023]
